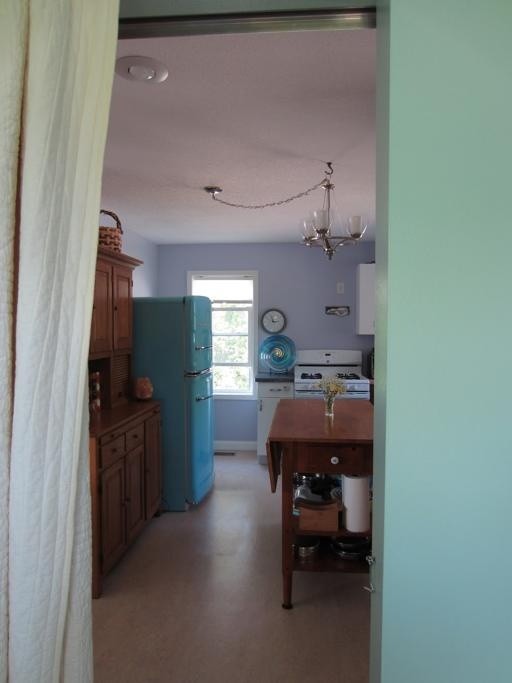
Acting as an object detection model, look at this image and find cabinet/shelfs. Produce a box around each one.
[86,250,137,360]
[136,399,165,522]
[256,376,294,463]
[353,260,377,337]
[89,414,146,599]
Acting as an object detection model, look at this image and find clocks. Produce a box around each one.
[260,308,287,334]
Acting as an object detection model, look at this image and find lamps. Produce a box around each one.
[300,160,367,260]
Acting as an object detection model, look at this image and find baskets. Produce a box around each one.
[99,209,123,253]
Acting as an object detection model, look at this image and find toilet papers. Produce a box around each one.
[341,473,370,532]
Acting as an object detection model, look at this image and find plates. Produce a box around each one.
[292,535,371,565]
[259,334,297,372]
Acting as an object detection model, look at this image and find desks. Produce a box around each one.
[264,395,374,610]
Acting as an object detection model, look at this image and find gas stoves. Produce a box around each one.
[294,371,370,392]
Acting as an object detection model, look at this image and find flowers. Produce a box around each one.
[313,374,348,416]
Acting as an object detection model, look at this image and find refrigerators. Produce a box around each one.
[128,294,215,513]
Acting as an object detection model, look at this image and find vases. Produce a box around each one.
[320,390,338,416]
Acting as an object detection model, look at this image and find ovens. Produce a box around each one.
[294,391,370,399]
